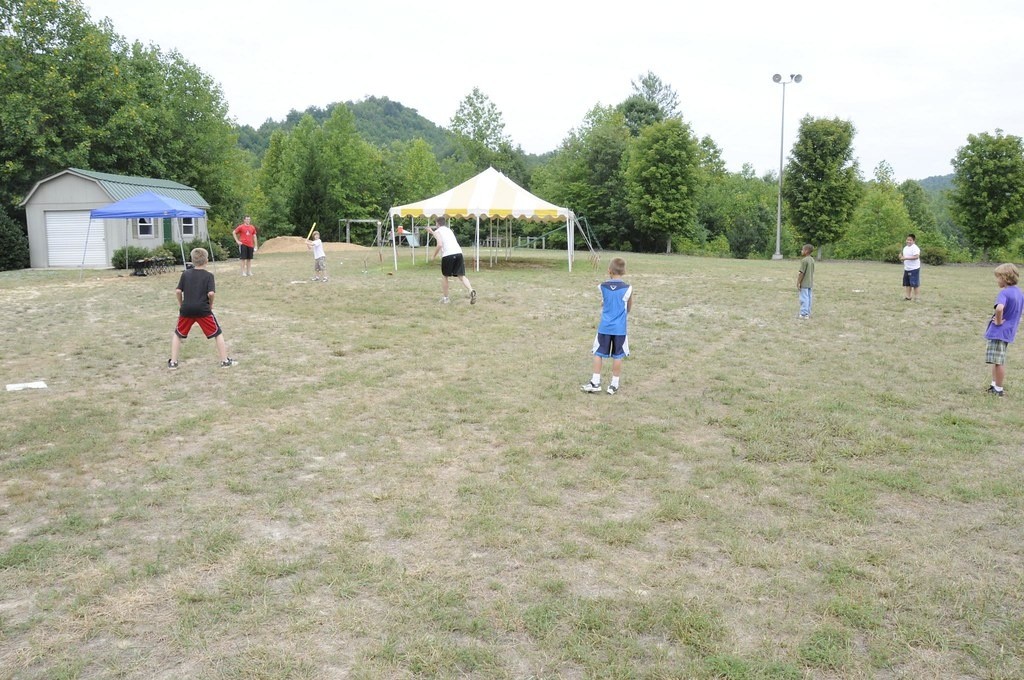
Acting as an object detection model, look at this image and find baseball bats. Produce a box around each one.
[306,222,317,240]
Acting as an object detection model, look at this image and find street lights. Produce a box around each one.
[772,72,802,260]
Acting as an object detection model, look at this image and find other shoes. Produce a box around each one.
[438,297,449,303]
[221,357,238,369]
[246,272,254,276]
[167,359,179,368]
[796,314,809,320]
[470,289,477,305]
[312,275,320,280]
[242,273,247,277]
[902,297,912,301]
[321,277,327,282]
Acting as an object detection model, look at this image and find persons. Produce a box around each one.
[984,263,1024,397]
[796,244,814,319]
[425,217,477,305]
[899,234,921,302]
[167,248,238,370]
[233,216,257,277]
[305,231,328,282]
[580,258,633,395]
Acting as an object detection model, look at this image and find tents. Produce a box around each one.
[390,167,575,272]
[80,192,216,280]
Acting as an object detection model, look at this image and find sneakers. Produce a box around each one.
[580,381,602,392]
[981,386,1003,398]
[607,385,619,394]
